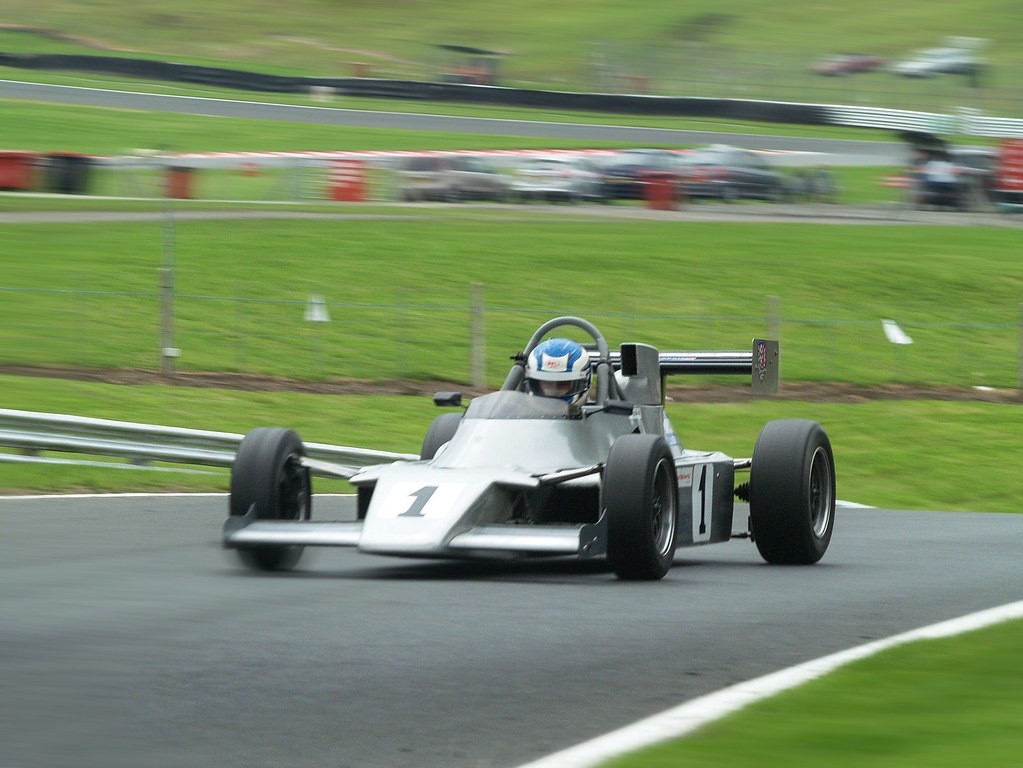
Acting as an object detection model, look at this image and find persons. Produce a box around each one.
[522,338,593,419]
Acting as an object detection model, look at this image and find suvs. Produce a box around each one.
[382,42,1007,208]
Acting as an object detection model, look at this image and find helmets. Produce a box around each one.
[526,336,593,417]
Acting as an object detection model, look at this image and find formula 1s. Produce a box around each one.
[222,315,839,583]
